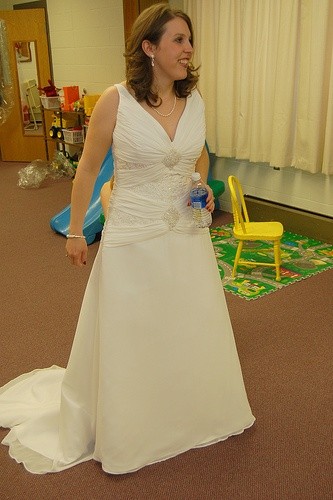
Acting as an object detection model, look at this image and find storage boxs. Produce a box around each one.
[39,95,62,109]
[62,129,84,144]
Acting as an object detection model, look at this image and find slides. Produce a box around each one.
[49,124,115,246]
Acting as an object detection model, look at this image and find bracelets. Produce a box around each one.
[66,235,85,239]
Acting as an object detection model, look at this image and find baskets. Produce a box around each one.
[62,129,82,143]
[39,96,60,109]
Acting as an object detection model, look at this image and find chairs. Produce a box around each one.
[228,174,284,282]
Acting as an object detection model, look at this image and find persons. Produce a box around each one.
[0,3,255,474]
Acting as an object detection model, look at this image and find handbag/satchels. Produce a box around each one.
[84,94,102,116]
[63,86,79,111]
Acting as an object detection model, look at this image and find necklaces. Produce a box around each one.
[151,95,177,116]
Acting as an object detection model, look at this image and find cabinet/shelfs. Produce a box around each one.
[38,105,90,166]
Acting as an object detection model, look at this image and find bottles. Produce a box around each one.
[191,172,212,228]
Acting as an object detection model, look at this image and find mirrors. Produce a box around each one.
[13,40,45,137]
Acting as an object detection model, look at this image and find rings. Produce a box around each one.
[66,254,69,256]
